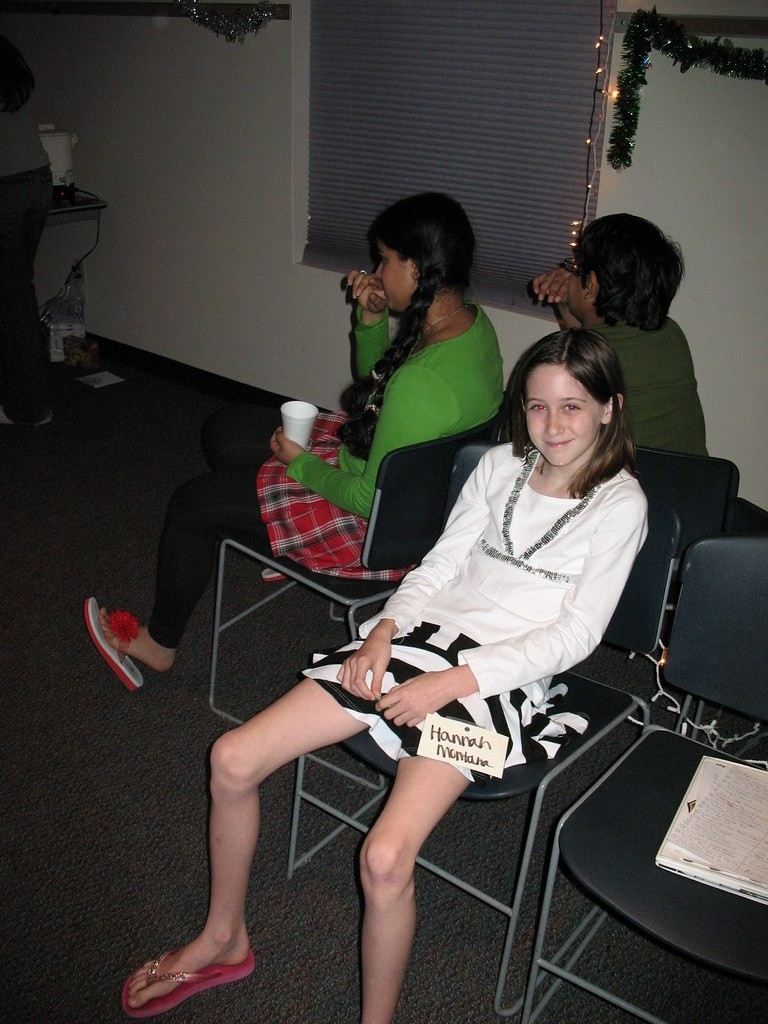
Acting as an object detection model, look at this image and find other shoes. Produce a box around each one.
[1,405,52,426]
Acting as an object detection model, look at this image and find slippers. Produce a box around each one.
[260,569,284,582]
[121,946,255,1019]
[84,597,144,692]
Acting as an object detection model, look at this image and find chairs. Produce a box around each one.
[209,390,767,1024]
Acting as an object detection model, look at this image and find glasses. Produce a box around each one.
[563,257,588,273]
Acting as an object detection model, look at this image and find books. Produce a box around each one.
[655,756,768,906]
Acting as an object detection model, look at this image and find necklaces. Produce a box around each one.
[408,306,467,350]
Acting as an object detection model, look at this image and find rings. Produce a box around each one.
[359,270,368,275]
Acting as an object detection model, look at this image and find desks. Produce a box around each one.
[46,194,107,225]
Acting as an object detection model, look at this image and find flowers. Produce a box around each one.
[105,608,140,641]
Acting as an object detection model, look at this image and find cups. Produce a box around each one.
[280,401,319,449]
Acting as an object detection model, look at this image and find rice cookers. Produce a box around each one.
[37,124,76,202]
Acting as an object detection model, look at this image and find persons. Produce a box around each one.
[83,191,506,692]
[0,30,57,428]
[120,327,650,1023]
[532,213,709,457]
